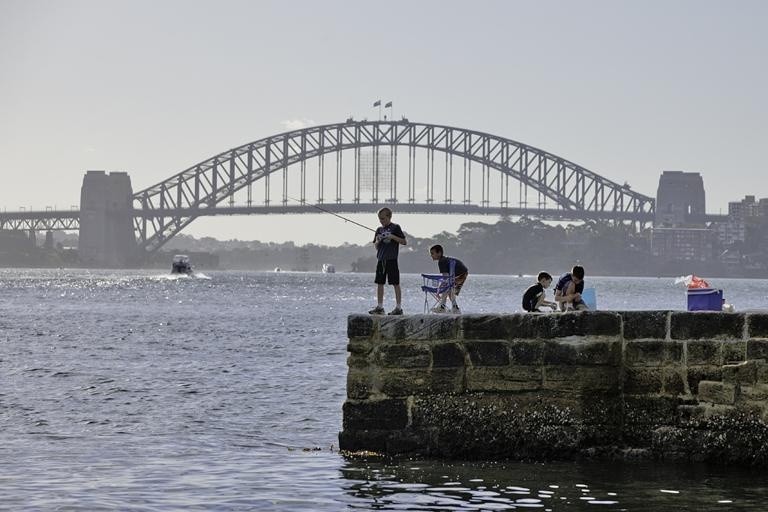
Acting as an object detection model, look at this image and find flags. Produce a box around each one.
[372,99,393,108]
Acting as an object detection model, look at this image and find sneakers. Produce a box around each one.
[368,306,385,315]
[387,306,403,316]
[528,308,542,313]
[430,303,461,314]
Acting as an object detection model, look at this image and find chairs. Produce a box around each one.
[421,261,457,315]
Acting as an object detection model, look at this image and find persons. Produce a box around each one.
[369,206,468,316]
[522,264,591,312]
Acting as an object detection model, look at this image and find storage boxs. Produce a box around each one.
[687,289,723,310]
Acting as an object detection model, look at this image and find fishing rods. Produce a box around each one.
[282,194,388,237]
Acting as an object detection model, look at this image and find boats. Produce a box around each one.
[322,263,336,274]
[171,255,192,275]
